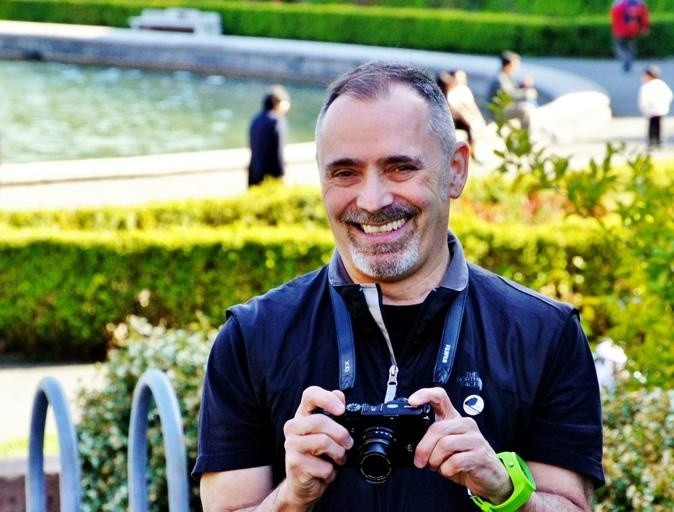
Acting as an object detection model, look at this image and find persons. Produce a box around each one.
[190,59,606,512]
[247,85,290,186]
[636,64,673,153]
[435,49,538,167]
[609,0,649,74]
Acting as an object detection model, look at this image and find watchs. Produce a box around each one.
[467,450,537,512]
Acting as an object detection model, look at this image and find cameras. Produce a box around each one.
[324,397,436,486]
